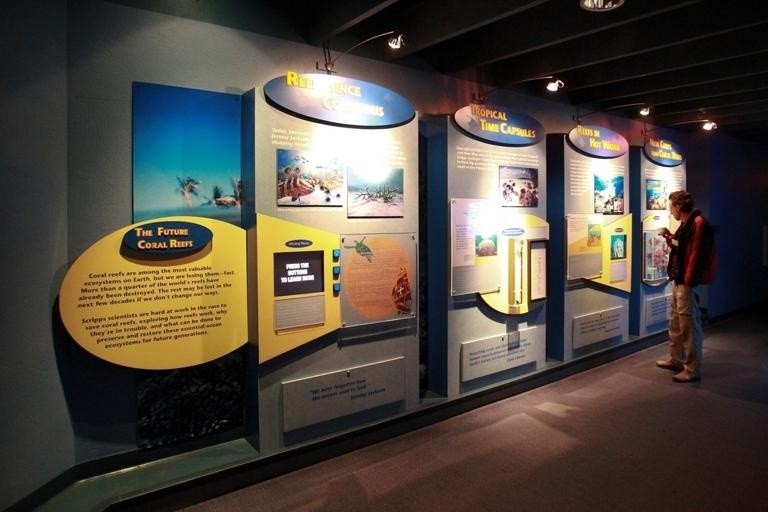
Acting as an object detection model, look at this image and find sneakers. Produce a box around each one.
[671,368,702,382]
[656,356,684,370]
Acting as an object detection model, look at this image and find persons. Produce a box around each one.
[654,189,717,384]
[501,179,537,206]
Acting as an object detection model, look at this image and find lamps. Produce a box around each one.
[641,108,718,134]
[575,101,652,126]
[326,31,408,74]
[476,74,572,107]
